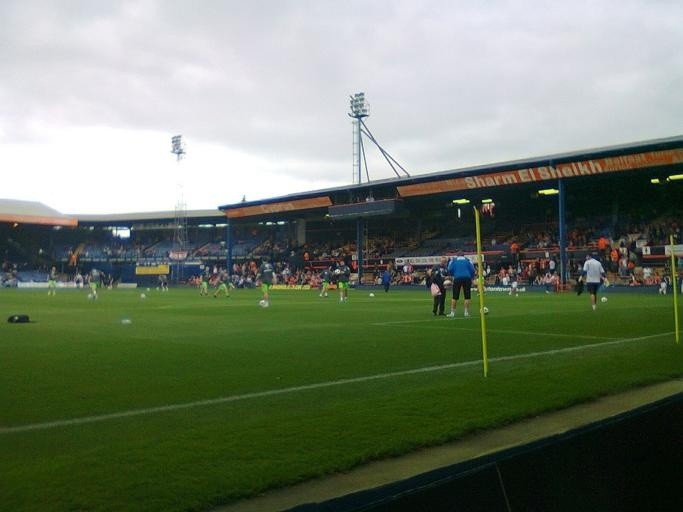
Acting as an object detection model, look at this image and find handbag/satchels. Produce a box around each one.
[430,282,442,296]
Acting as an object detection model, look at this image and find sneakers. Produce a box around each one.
[318,291,349,302]
[433,309,472,318]
[261,300,270,309]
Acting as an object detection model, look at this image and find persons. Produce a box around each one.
[431,257,454,316]
[0,219,683,301]
[447,252,475,317]
[256,257,276,307]
[579,253,607,310]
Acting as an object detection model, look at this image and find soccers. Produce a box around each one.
[146,287,150,292]
[259,300,265,306]
[480,307,488,315]
[88,294,93,300]
[370,293,374,297]
[601,297,607,302]
[156,287,160,291]
[141,294,146,299]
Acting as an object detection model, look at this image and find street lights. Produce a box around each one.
[347,91,369,182]
[171,134,186,211]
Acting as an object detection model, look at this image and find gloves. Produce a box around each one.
[603,278,610,288]
